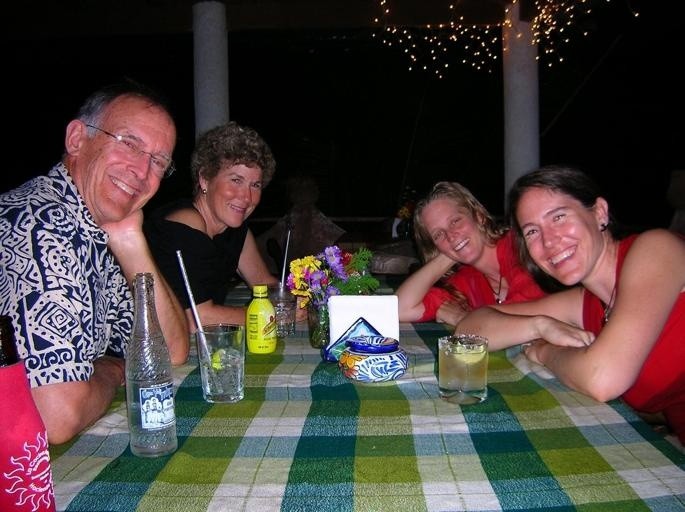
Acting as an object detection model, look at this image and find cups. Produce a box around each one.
[436,333,490,406]
[270,290,299,338]
[195,323,246,406]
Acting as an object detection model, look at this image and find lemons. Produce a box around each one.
[451,341,486,364]
[211,349,226,370]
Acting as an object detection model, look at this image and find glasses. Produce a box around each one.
[85,126,177,180]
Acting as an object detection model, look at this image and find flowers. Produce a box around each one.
[284,243,380,339]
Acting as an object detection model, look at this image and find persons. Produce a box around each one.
[378,179,561,323]
[448,162,684,445]
[0,88,192,445]
[131,119,315,334]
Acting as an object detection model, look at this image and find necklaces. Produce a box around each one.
[603,285,616,324]
[481,269,505,304]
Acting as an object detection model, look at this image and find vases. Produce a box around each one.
[306,304,329,347]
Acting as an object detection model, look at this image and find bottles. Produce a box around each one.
[245,283,278,355]
[124,271,180,460]
